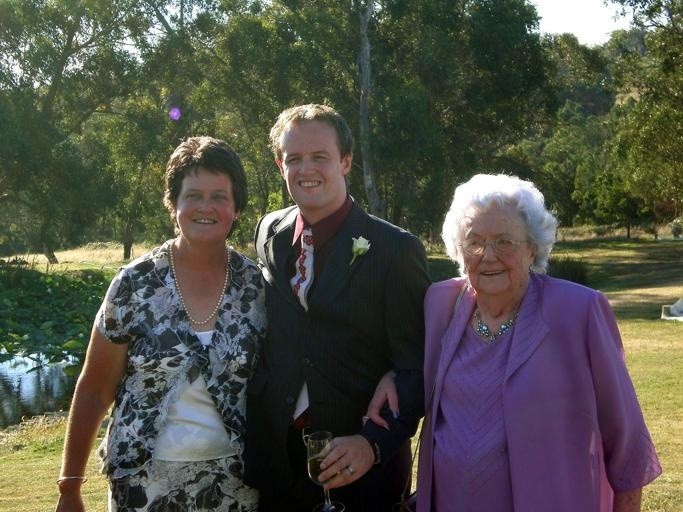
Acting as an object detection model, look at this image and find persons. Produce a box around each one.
[357,168,663,512]
[251,102,432,512]
[50,136,269,512]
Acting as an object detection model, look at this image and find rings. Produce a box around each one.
[345,465,353,475]
[361,415,369,421]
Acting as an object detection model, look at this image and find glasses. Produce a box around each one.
[456,238,531,257]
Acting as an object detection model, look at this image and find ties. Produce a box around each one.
[285,230,314,421]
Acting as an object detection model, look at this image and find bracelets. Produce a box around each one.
[53,474,88,486]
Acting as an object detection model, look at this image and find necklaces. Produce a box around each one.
[167,238,231,326]
[469,308,518,345]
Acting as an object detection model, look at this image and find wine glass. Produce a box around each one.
[307,431,345,511]
